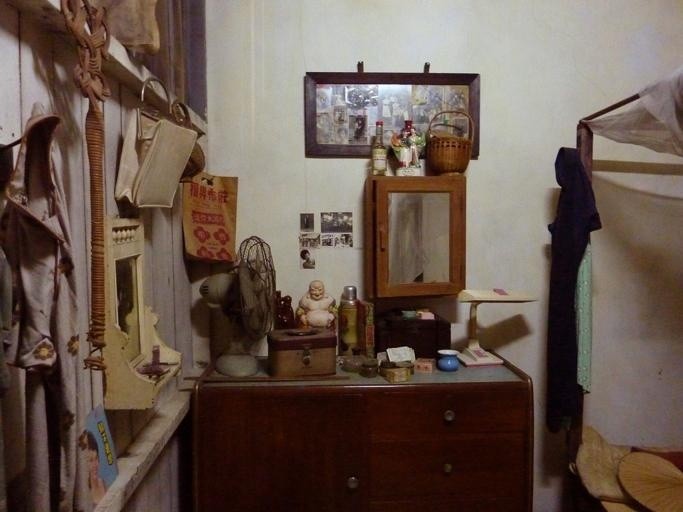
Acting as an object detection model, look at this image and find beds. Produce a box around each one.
[558,67,683,512]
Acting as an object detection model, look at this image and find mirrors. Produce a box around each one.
[302,68,482,163]
[379,184,456,292]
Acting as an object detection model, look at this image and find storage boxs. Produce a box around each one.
[265,324,338,379]
[372,308,453,361]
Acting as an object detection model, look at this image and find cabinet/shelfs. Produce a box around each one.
[359,171,471,308]
[189,345,536,512]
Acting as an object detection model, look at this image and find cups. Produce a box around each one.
[437,349,458,371]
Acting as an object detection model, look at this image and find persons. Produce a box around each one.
[295,280,336,329]
[399,127,419,167]
[381,92,401,128]
[336,127,348,145]
[86,431,108,507]
[301,249,316,269]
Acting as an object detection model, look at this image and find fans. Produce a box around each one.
[197,231,283,380]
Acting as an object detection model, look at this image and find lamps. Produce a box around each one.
[454,283,541,368]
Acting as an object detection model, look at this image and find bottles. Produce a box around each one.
[338,285,359,354]
[400,120,416,141]
[371,120,388,176]
